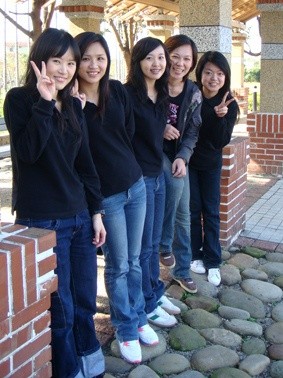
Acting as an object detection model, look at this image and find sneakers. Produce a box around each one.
[173,275,198,293]
[138,325,159,345]
[147,306,177,327]
[159,252,176,268]
[119,339,142,364]
[157,295,181,314]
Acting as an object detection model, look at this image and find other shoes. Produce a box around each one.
[207,268,221,286]
[190,260,206,275]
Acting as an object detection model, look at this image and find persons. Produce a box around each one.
[191,50,239,287]
[69,31,159,364]
[3,28,107,378]
[122,37,181,328]
[159,34,204,293]
